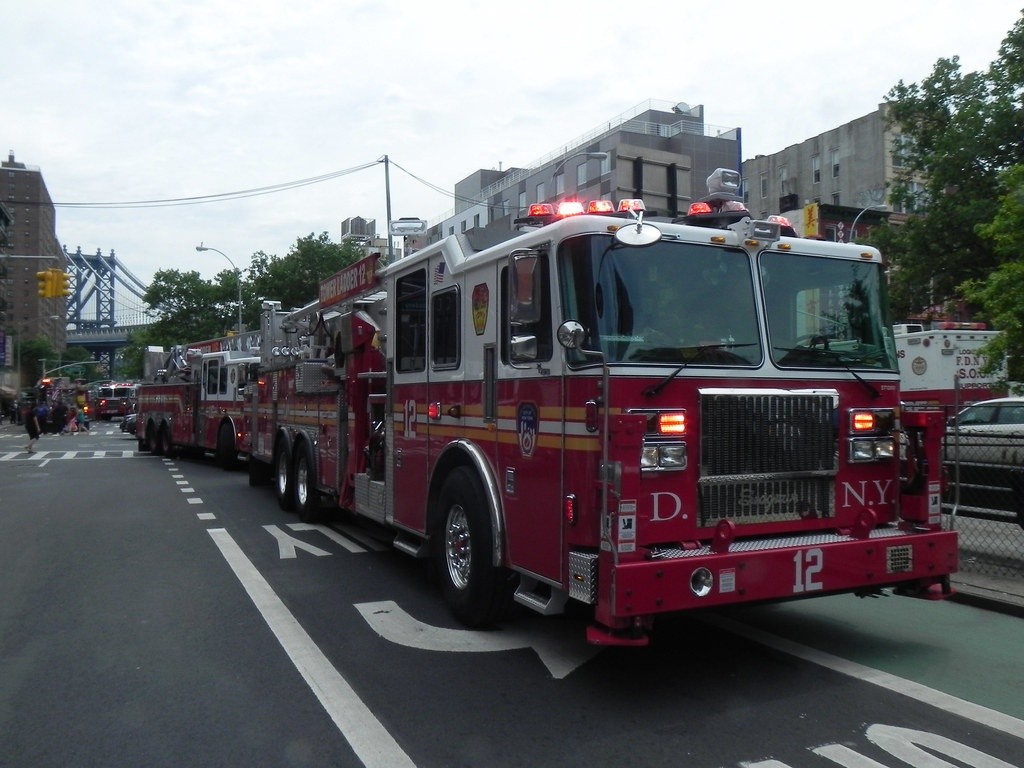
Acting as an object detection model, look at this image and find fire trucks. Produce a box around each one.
[244,168,962,674]
[815,322,1010,430]
[134,330,262,472]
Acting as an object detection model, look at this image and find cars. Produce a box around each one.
[66,380,143,421]
[899,396,1023,472]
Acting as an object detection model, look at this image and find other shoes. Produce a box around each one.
[25,446,33,453]
[39,433,44,437]
[52,433,60,437]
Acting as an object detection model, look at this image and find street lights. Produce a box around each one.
[545,152,608,204]
[849,204,888,244]
[196,247,242,335]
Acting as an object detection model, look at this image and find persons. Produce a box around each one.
[77,409,90,436]
[19,399,69,454]
[9,398,32,425]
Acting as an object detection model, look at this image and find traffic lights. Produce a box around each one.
[56,269,70,298]
[36,271,52,298]
